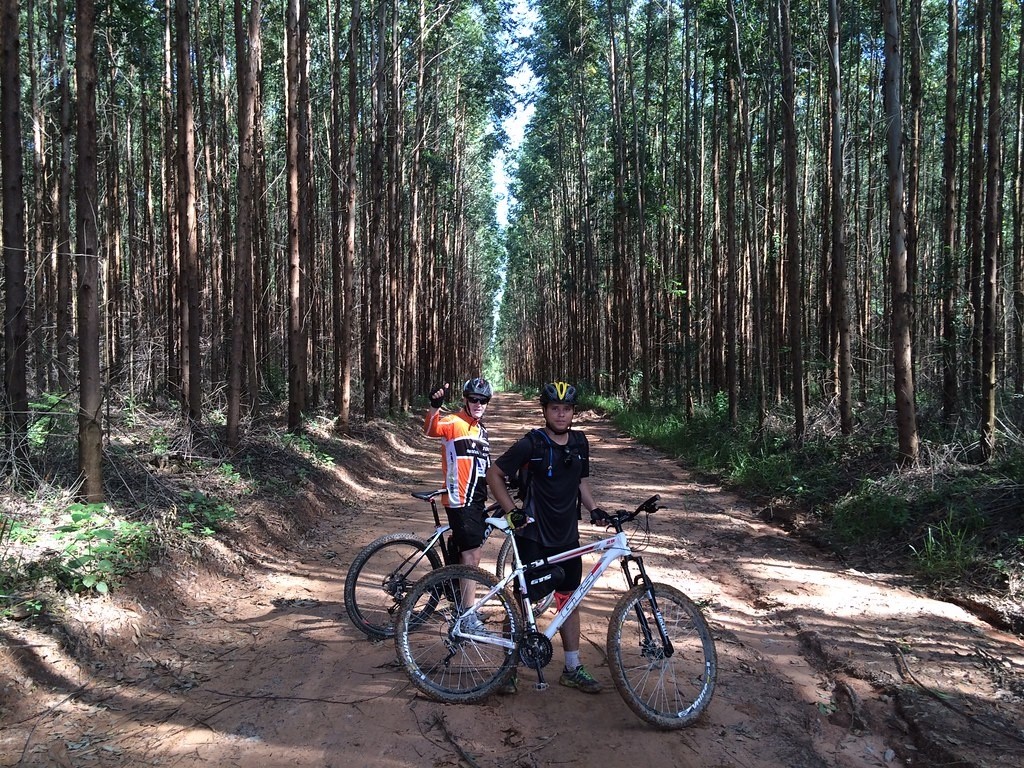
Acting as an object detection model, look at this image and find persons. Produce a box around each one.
[423,377,499,638]
[486,381,609,694]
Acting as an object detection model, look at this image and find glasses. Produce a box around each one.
[466,396,488,404]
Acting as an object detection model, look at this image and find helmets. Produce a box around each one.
[463,377,492,399]
[540,381,577,405]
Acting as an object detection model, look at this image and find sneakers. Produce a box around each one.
[560,665,603,693]
[501,676,517,693]
[464,620,495,637]
[475,609,491,619]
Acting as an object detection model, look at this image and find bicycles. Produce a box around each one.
[344,475,556,639]
[395,494,718,729]
